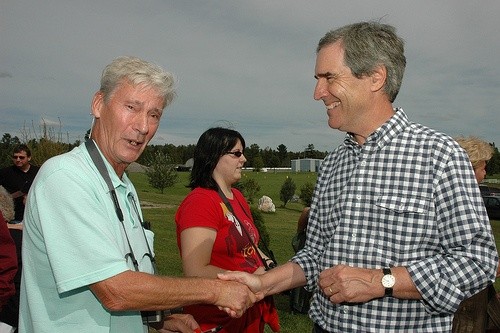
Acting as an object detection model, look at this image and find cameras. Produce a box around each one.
[141,307,172,323]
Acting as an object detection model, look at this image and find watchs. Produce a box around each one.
[382,267,395,296]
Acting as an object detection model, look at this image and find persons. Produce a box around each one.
[0,143,40,333]
[18,55,256,333]
[453,137,494,184]
[174,127,280,333]
[217,21,499,333]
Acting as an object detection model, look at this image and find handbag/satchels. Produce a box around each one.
[451,280,500,333]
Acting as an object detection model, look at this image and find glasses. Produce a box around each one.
[13,156,25,159]
[222,151,244,157]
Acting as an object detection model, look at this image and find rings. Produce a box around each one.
[329,286,334,296]
